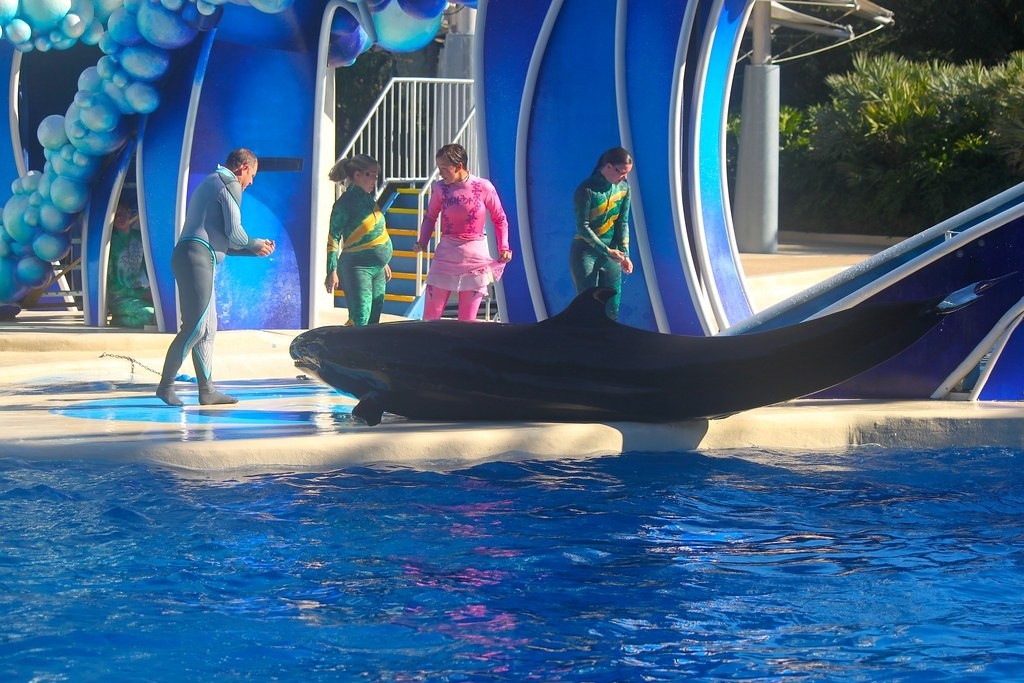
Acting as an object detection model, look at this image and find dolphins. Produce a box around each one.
[289,270,1019,426]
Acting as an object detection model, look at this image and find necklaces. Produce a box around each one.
[603,187,613,210]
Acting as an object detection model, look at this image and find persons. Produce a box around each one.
[156,148,276,407]
[570,148,634,319]
[324,154,394,327]
[413,143,513,321]
